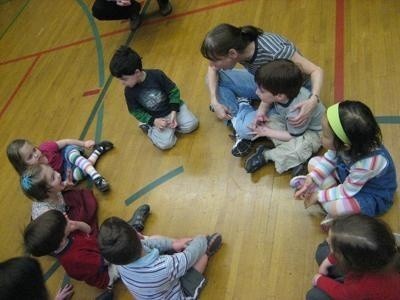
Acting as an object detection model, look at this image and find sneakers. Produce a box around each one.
[138,123,149,132]
[294,163,307,174]
[290,176,307,188]
[206,233,222,257]
[231,137,252,157]
[127,204,150,232]
[245,143,266,173]
[320,216,334,232]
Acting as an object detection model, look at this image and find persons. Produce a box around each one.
[244,58,327,179]
[0,256,74,300]
[109,45,199,150]
[6,138,114,192]
[290,99,398,233]
[22,203,150,290]
[305,211,400,300]
[20,163,98,239]
[92,0,172,30]
[97,216,222,300]
[200,23,324,157]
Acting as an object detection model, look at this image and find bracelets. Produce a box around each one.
[209,103,215,113]
[309,94,320,104]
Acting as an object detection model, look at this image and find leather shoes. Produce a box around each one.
[94,176,109,192]
[160,2,172,16]
[130,16,139,29]
[94,141,113,154]
